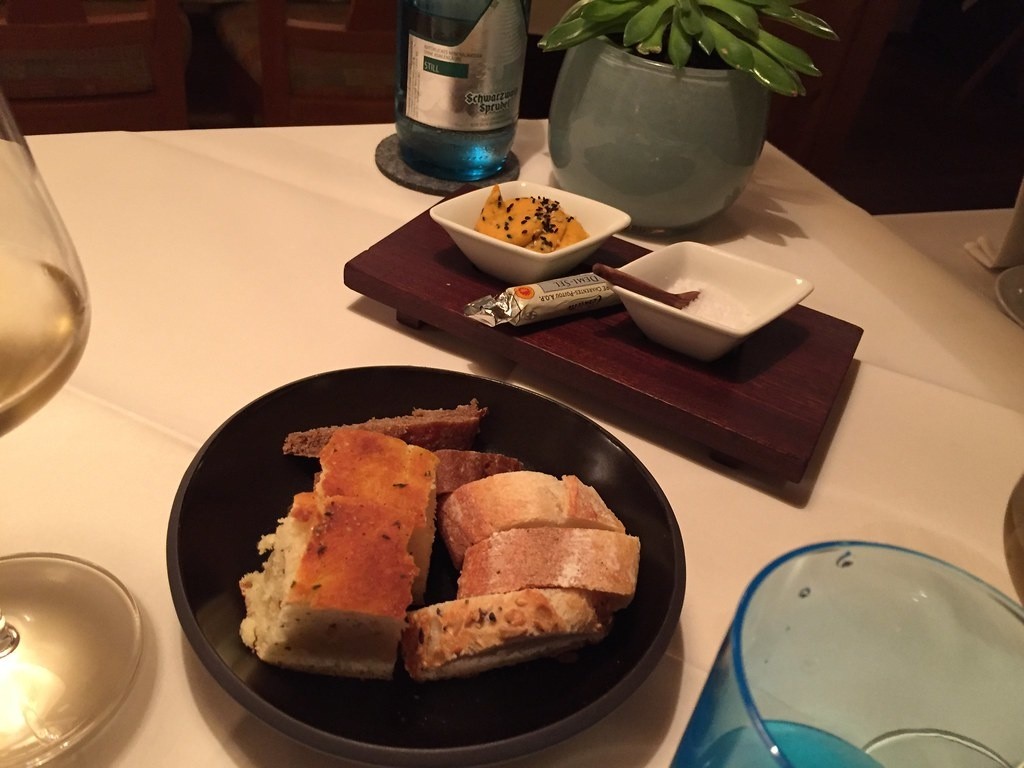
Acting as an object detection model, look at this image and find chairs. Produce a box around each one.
[197,0,397,129]
[0,0,196,141]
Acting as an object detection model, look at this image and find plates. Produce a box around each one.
[168,354,687,768]
[996,265,1022,329]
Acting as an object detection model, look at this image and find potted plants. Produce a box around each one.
[533,0,845,230]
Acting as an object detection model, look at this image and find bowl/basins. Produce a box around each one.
[611,239,813,363]
[424,181,632,283]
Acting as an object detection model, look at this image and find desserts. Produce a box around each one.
[238,399,642,681]
[475,184,591,254]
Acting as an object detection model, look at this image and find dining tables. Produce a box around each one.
[1,114,1023,767]
[871,205,1024,334]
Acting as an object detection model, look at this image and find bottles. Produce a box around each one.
[1,88,91,437]
[395,0,529,184]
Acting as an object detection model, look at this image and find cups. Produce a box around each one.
[672,539,1022,768]
